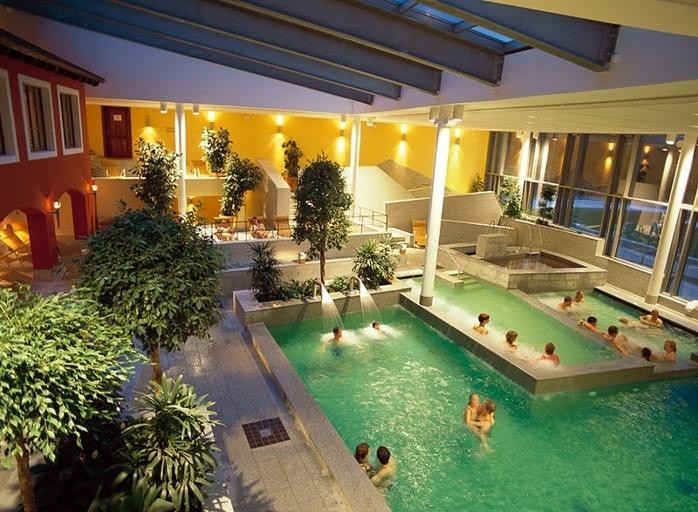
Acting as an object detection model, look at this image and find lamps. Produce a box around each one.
[160,104,167,114]
[340,129,344,137]
[455,138,461,144]
[208,122,215,131]
[664,131,678,146]
[341,114,346,122]
[277,125,283,133]
[49,200,63,228]
[192,105,200,116]
[513,128,561,143]
[88,183,100,232]
[145,120,152,132]
[401,134,407,142]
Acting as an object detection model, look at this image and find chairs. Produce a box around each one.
[0,222,87,284]
[410,219,429,246]
[211,214,293,245]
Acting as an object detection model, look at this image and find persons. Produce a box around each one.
[354,442,375,479]
[574,290,585,304]
[536,341,560,367]
[503,330,519,352]
[472,312,490,335]
[577,319,637,357]
[476,397,496,434]
[559,295,573,309]
[214,219,234,241]
[638,347,651,361]
[372,321,379,329]
[587,316,597,326]
[328,326,343,342]
[639,309,664,328]
[249,217,266,239]
[370,446,396,487]
[465,392,494,455]
[661,339,677,364]
